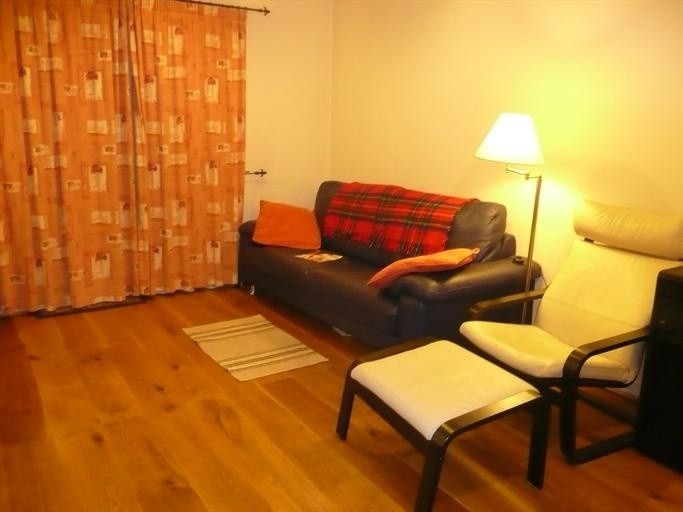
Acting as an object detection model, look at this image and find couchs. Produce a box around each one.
[238,182,542,352]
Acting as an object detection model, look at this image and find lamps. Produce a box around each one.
[476,111,543,325]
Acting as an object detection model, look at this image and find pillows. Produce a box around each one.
[366,247,480,289]
[252,200,321,253]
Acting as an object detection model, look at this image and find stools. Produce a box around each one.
[336,336,551,511]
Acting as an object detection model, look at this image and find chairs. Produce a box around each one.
[456,198,683,466]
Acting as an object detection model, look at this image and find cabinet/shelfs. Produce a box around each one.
[633,266,683,473]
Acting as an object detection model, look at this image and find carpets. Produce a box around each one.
[180,313,330,383]
